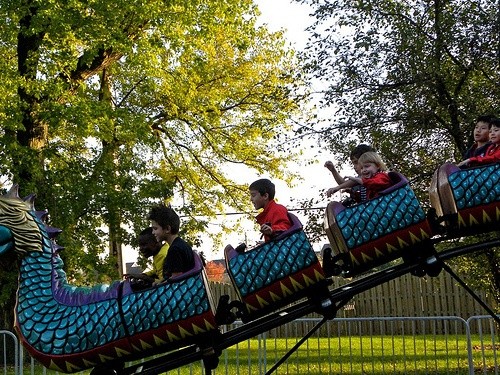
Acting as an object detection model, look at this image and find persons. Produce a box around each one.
[249,179,292,242]
[324,116,500,203]
[122,228,170,286]
[147,203,196,286]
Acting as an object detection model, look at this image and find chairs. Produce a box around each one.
[171,171,409,283]
